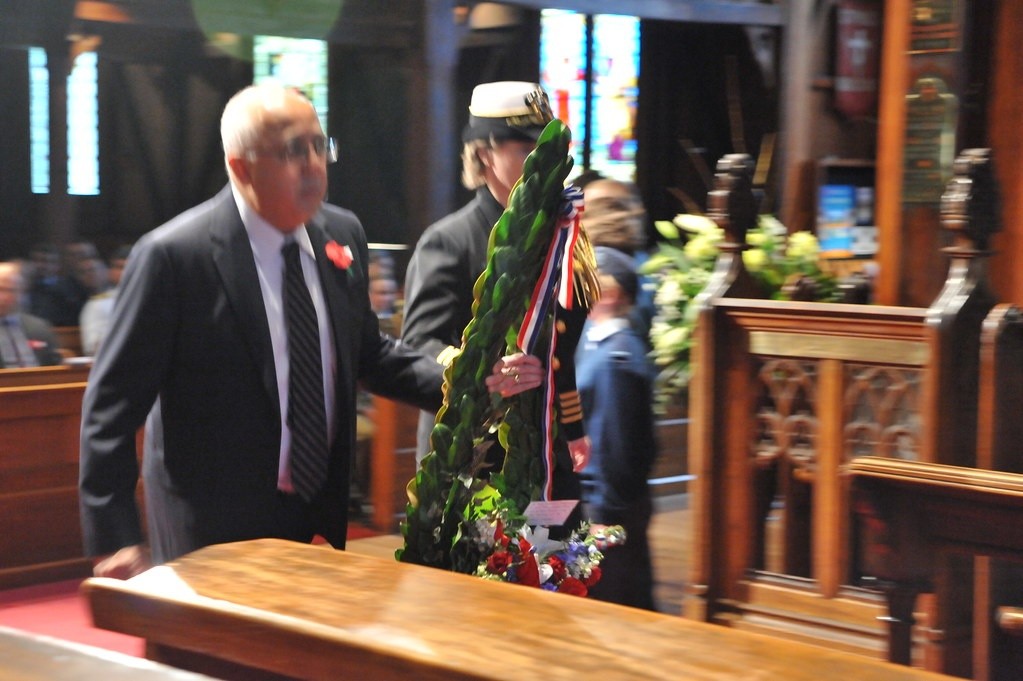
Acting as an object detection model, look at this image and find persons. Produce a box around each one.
[0,239,397,372]
[398,79,603,545]
[78,77,543,679]
[572,244,658,612]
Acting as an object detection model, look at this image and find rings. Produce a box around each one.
[514,373,520,384]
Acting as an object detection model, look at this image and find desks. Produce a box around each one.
[80,539,971,681]
[46,327,85,360]
[0,362,153,592]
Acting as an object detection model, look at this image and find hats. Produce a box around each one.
[461,81,554,142]
[593,245,638,302]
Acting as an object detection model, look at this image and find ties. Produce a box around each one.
[3,321,25,367]
[280,233,329,504]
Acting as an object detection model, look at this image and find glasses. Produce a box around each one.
[247,135,327,159]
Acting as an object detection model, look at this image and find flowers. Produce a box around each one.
[393,118,628,598]
